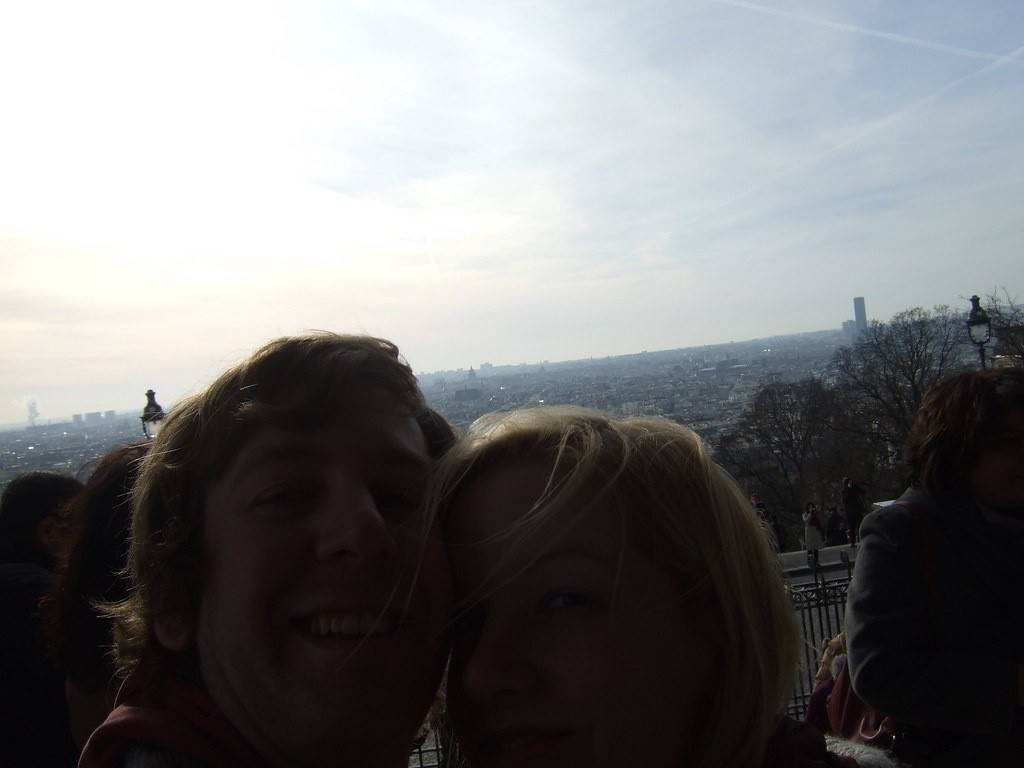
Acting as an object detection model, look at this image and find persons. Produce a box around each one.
[0,329,1024,768]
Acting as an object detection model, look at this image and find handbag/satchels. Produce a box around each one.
[826,662,897,749]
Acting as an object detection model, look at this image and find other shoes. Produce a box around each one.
[850,545,857,548]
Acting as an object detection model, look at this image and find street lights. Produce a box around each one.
[965,294,992,368]
